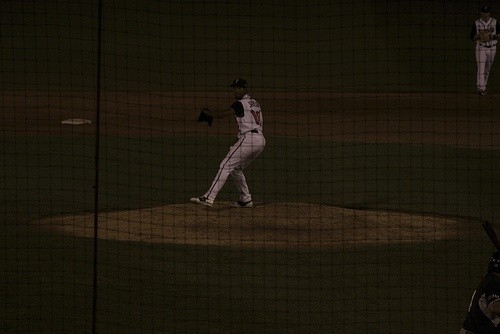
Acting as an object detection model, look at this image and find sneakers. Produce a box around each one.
[230,200,253,208]
[190,197,214,208]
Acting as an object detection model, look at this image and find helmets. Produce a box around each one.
[480,6,489,12]
[228,77,248,88]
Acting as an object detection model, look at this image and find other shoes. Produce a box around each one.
[480,92,489,99]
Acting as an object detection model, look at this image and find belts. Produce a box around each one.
[251,130,258,133]
[479,44,496,48]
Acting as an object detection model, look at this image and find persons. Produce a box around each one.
[470,6,500,96]
[461,251,500,334]
[190,80,266,209]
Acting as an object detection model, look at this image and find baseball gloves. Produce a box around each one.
[479,29,494,43]
[195,108,214,128]
